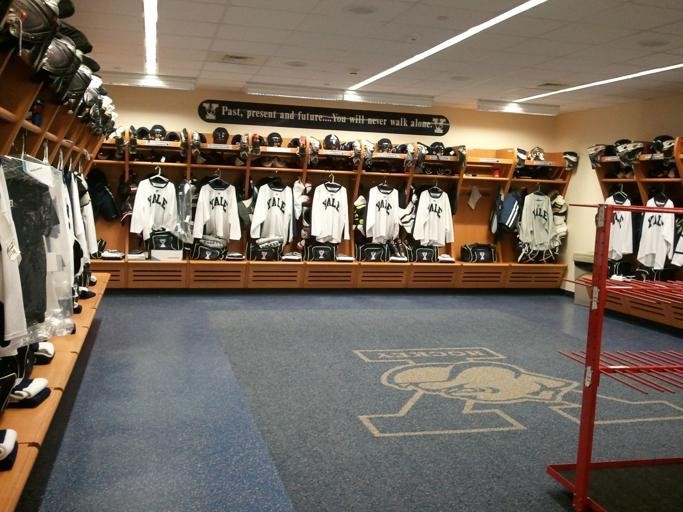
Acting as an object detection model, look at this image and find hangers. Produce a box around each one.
[613,183,628,199]
[150,166,442,191]
[533,184,545,196]
[654,184,668,200]
[0,133,83,179]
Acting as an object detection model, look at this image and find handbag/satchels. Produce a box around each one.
[460,243,497,262]
[247,239,283,261]
[302,243,336,261]
[144,231,183,250]
[609,261,632,279]
[189,235,229,260]
[405,241,439,262]
[635,265,673,281]
[356,240,389,262]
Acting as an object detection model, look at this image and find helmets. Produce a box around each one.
[324,134,340,150]
[231,135,241,144]
[378,139,392,151]
[430,142,456,156]
[288,139,299,148]
[529,146,545,160]
[563,151,577,170]
[341,141,352,150]
[268,133,283,146]
[253,134,264,145]
[517,149,527,160]
[136,125,182,141]
[198,133,206,142]
[395,145,407,153]
[213,127,228,143]
[588,134,677,163]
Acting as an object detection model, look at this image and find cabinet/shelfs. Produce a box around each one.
[546,204,683,512]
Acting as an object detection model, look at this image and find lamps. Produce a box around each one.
[475,98,560,118]
[95,70,197,92]
[246,83,343,104]
[344,91,433,108]
